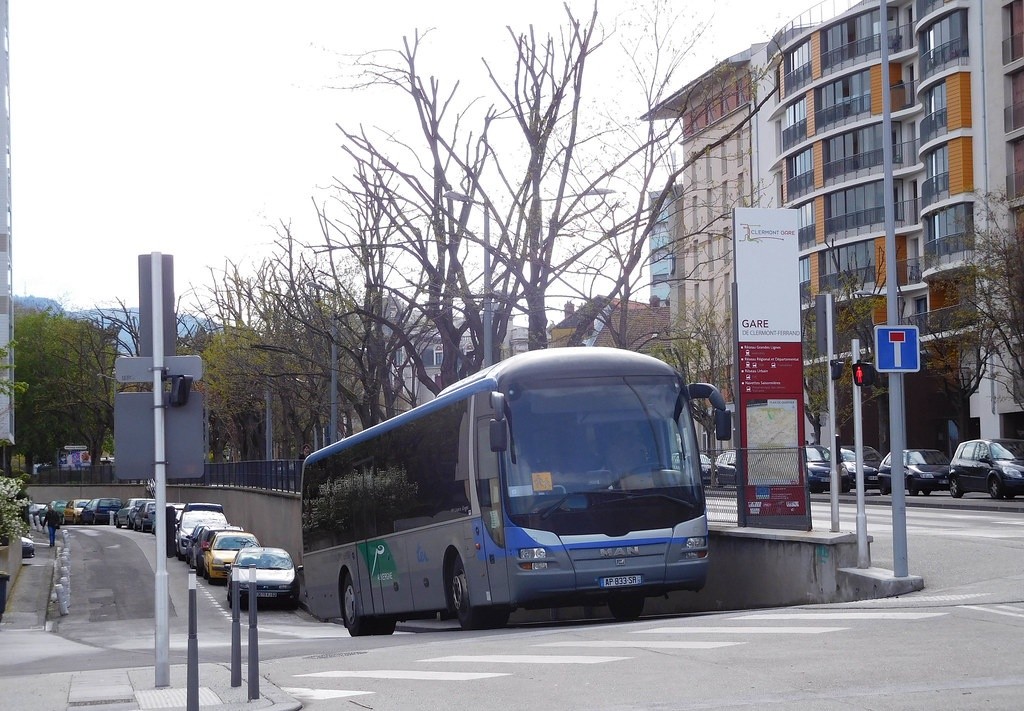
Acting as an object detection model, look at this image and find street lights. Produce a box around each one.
[306,282,338,444]
[442,190,492,367]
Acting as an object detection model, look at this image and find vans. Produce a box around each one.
[671,453,712,482]
[947,439,1024,499]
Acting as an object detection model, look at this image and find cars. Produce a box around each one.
[227,546,304,611]
[877,448,952,495]
[805,446,850,494]
[62,500,93,525]
[203,532,265,585]
[133,502,158,531]
[821,446,886,492]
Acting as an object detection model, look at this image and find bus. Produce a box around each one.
[301,345,731,641]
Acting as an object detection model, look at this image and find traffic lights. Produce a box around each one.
[851,363,875,388]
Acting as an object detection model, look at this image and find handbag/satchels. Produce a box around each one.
[54,524,60,530]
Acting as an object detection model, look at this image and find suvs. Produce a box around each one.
[28,501,69,523]
[179,510,231,560]
[153,502,225,541]
[114,498,156,529]
[78,498,124,526]
[186,524,245,575]
[714,449,737,487]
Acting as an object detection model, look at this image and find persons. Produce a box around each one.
[595,422,643,472]
[299,444,311,459]
[42,505,60,547]
